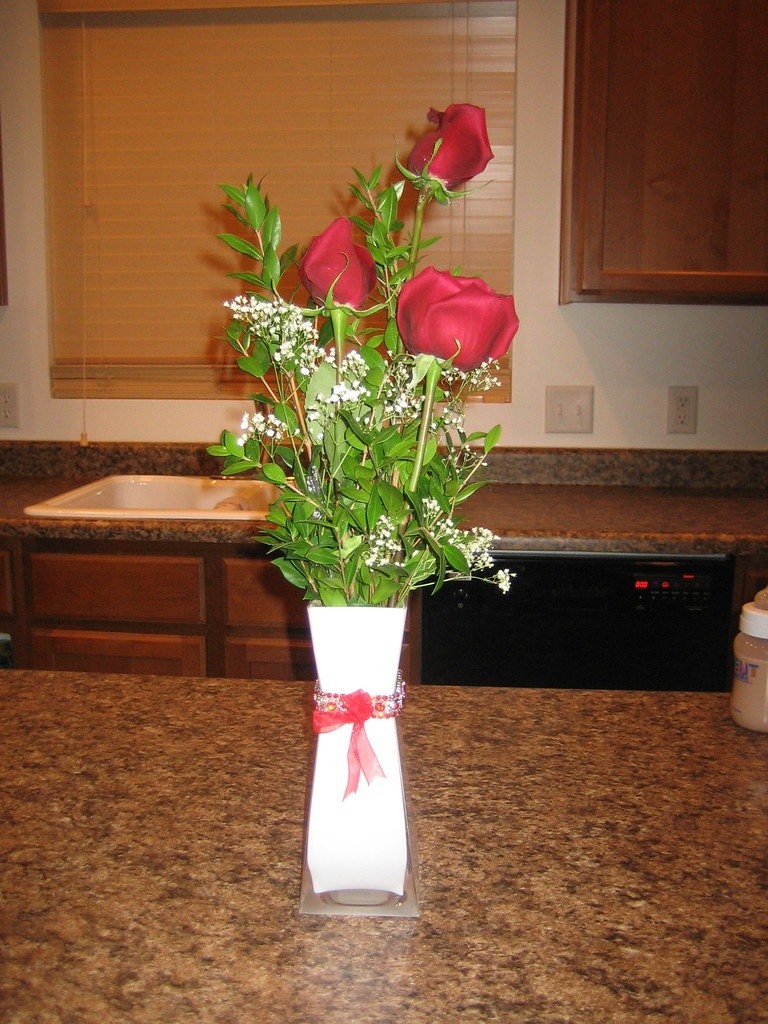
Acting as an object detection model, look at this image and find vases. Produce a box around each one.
[299,594,423,918]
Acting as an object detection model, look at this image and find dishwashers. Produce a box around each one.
[422,559,733,690]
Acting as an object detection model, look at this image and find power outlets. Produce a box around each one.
[0,382,20,429]
[667,385,698,434]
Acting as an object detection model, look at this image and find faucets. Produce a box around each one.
[253,391,299,475]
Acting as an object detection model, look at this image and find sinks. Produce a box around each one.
[23,472,286,521]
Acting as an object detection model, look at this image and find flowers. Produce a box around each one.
[204,103,520,608]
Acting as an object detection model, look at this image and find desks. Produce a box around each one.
[0,669,768,1024]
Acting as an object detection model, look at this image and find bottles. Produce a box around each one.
[731,586,768,733]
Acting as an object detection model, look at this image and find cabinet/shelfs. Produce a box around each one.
[0,437,418,686]
[557,0,768,307]
